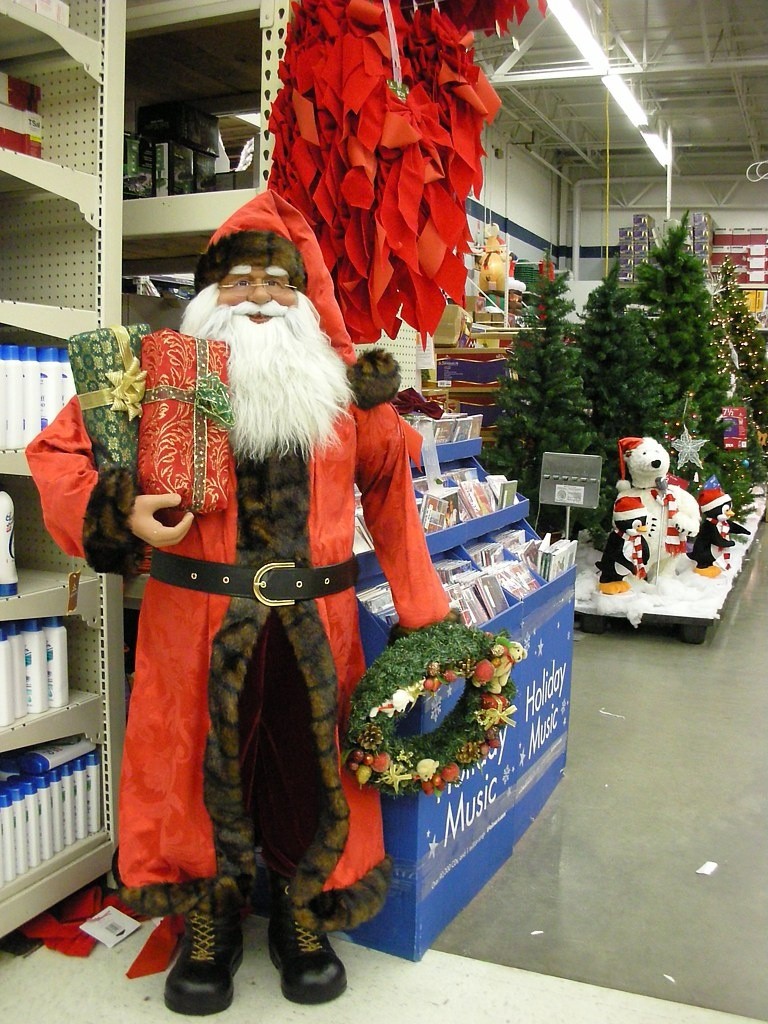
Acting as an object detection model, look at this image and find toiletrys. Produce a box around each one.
[0,345,77,452]
[0,615,70,729]
[0,490,19,597]
[0,735,101,894]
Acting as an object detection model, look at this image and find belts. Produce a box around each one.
[148,546,361,606]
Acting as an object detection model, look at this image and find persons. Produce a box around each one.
[26,190,454,1016]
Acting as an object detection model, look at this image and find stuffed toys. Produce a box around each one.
[595,436,751,595]
[400,758,440,787]
[472,641,528,694]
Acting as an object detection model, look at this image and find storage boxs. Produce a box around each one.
[192,151,216,193]
[136,101,219,158]
[234,171,253,189]
[618,212,768,283]
[215,172,234,191]
[724,407,748,451]
[153,139,193,196]
[123,134,156,199]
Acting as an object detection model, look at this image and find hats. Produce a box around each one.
[193,189,402,412]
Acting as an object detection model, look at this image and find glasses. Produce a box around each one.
[218,279,298,297]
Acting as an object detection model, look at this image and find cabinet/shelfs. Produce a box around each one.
[248,436,577,962]
[122,0,289,335]
[617,284,768,358]
[0,0,125,942]
